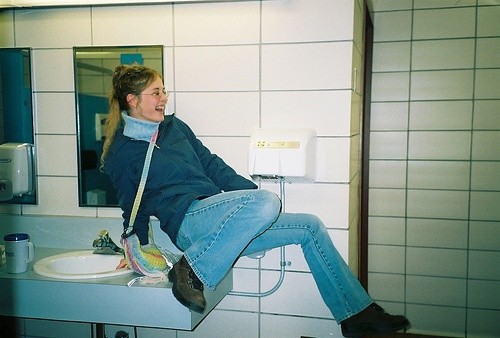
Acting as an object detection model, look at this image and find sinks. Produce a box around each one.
[32,249,134,280]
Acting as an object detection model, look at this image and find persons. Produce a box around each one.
[99,62,409,338]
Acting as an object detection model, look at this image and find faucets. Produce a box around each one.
[92,230,125,255]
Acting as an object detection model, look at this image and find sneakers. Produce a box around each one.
[340,302,410,338]
[168,255,206,313]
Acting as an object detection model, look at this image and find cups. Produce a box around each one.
[3,233,34,273]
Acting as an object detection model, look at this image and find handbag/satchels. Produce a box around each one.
[120,227,179,287]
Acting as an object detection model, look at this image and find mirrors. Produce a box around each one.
[73,44,164,207]
[0,47,38,206]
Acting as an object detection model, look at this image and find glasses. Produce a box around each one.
[132,90,170,98]
